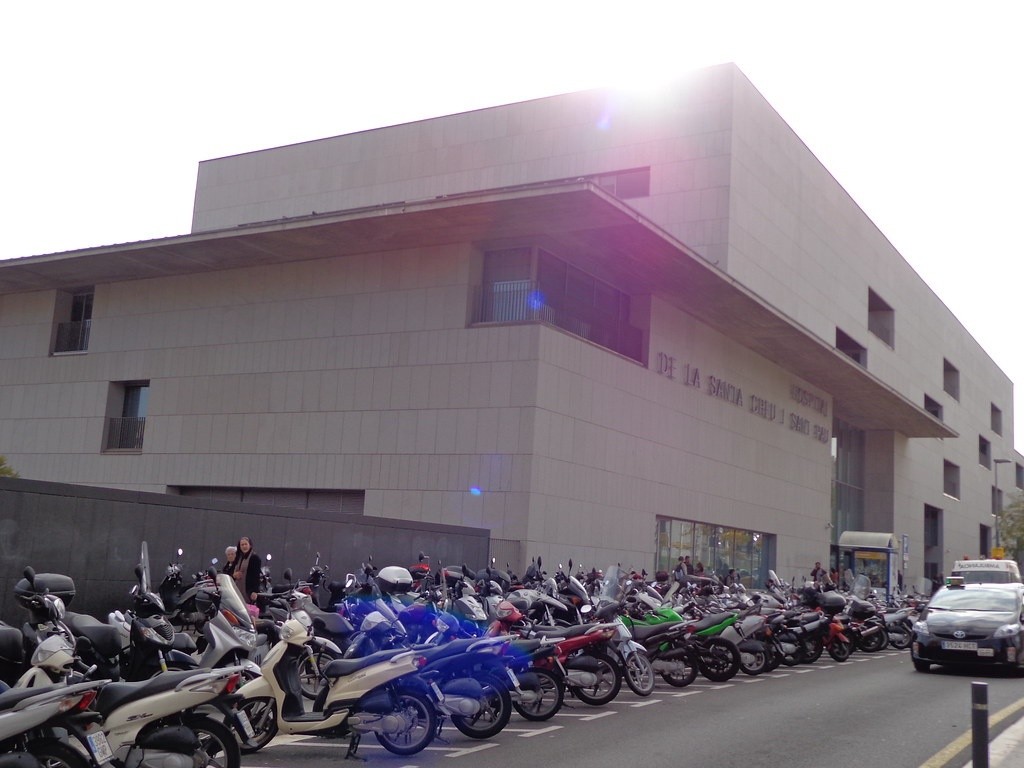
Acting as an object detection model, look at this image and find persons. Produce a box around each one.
[830,568,838,583]
[223,537,261,605]
[674,556,705,595]
[724,569,736,587]
[811,562,826,581]
[898,570,903,591]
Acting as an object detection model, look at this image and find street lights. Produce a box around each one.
[993,457,1014,546]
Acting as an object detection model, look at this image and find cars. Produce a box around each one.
[909,575,1024,678]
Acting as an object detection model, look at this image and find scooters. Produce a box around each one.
[0,534,935,768]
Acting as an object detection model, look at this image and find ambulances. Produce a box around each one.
[950,556,1023,613]
